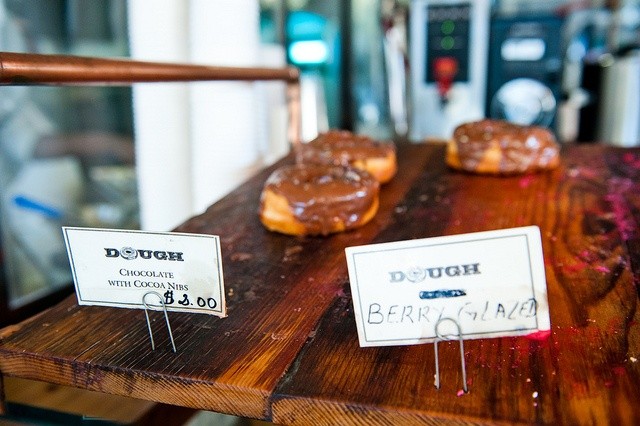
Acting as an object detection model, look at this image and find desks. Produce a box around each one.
[0,142,640,426]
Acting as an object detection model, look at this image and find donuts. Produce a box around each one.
[444,118,560,174]
[295,129,398,184]
[257,160,380,236]
